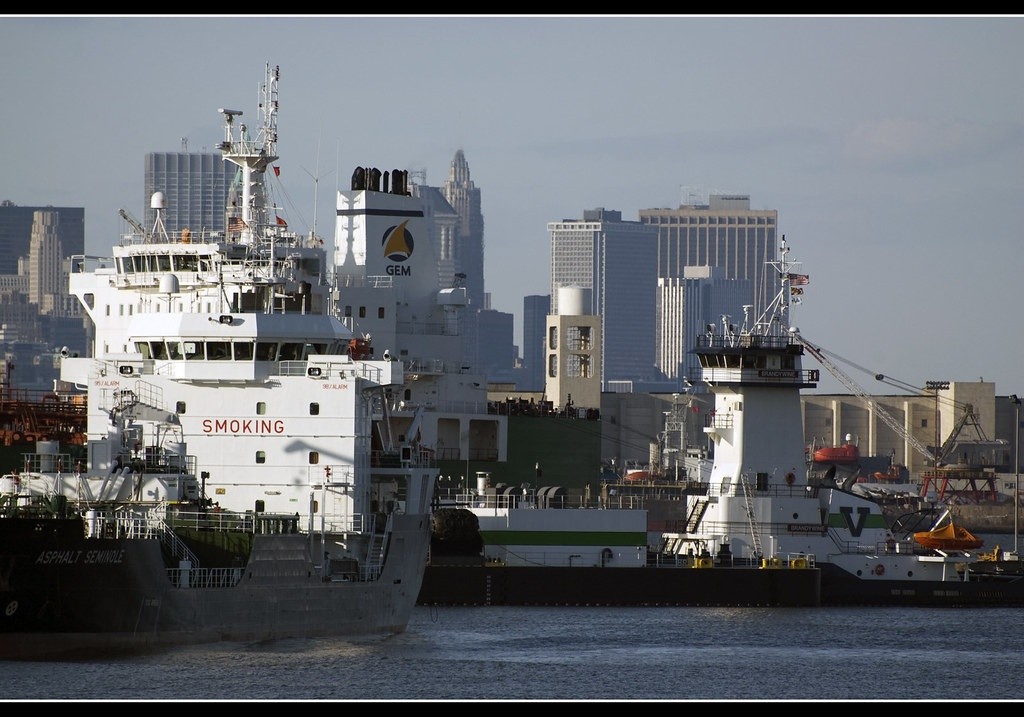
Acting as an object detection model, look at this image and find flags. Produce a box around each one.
[788,274,809,295]
[229,217,248,231]
[276,215,287,225]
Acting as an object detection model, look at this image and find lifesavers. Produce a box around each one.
[875,564,885,575]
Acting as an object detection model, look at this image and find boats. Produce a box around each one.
[913,522,984,551]
[415,234,1023,612]
[0,60,440,653]
[813,443,860,463]
